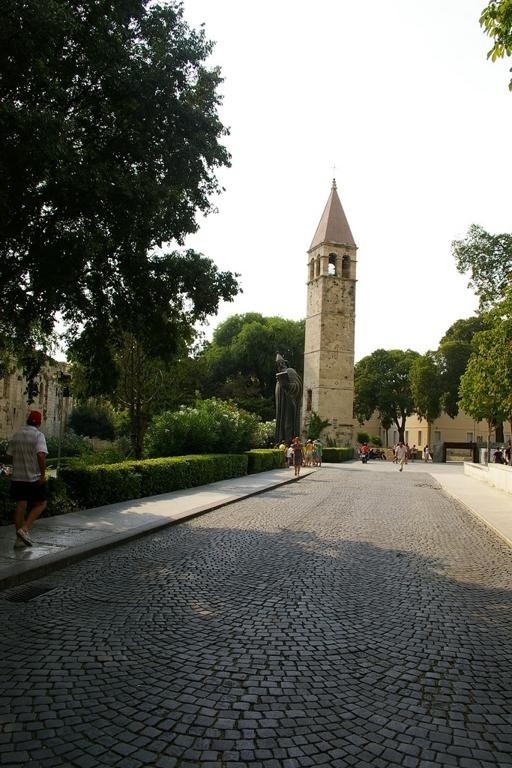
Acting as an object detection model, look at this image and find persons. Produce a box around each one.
[273,436,323,476]
[395,441,407,472]
[492,441,512,465]
[6,410,49,550]
[360,442,368,458]
[273,351,303,444]
[392,442,431,465]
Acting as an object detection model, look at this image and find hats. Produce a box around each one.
[28,411,41,423]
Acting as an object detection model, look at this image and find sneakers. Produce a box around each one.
[13,527,33,549]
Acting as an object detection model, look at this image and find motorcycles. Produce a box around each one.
[360,454,368,464]
[370,449,387,460]
